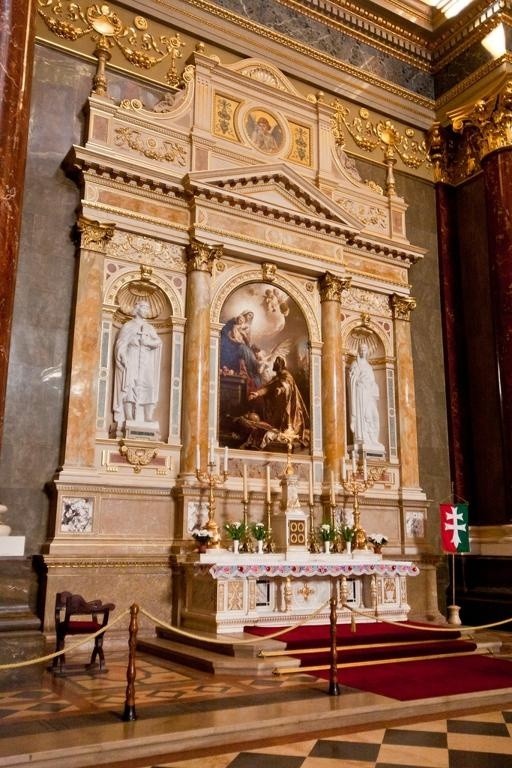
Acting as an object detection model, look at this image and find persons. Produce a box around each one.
[347,343,385,451]
[219,285,309,452]
[115,301,163,425]
[251,115,280,155]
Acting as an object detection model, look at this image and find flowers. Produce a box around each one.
[337,525,359,541]
[224,519,247,538]
[189,528,212,545]
[251,522,272,540]
[367,534,389,546]
[318,522,337,541]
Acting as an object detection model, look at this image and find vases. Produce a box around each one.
[324,541,330,555]
[257,541,263,554]
[233,539,239,553]
[199,544,207,553]
[346,541,353,554]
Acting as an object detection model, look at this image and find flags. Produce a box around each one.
[439,504,470,552]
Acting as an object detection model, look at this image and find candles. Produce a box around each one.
[195,439,375,507]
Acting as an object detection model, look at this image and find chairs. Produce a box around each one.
[52,590,113,676]
